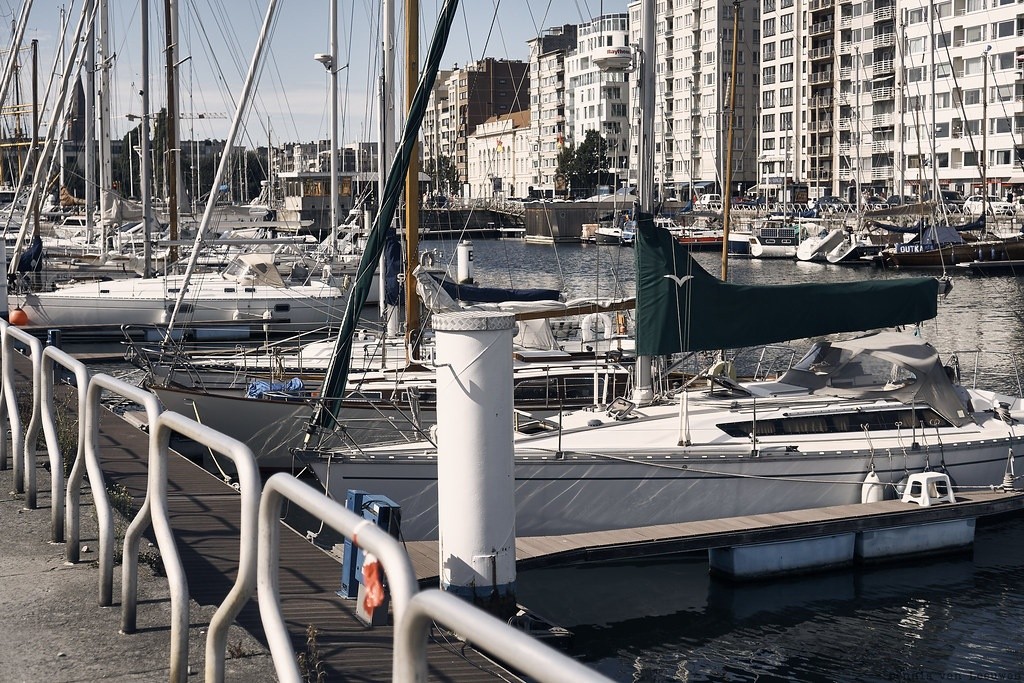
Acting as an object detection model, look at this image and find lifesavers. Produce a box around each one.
[578,313,613,344]
[420,250,436,269]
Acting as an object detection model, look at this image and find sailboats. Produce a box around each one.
[0,0,1024,580]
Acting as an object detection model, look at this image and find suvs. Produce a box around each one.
[923,189,966,215]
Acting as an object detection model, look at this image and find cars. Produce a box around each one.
[818,196,855,213]
[861,196,888,210]
[962,194,1017,216]
[886,193,916,208]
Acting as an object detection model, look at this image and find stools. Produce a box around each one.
[901,471,957,507]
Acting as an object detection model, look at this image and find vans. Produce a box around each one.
[695,193,722,210]
